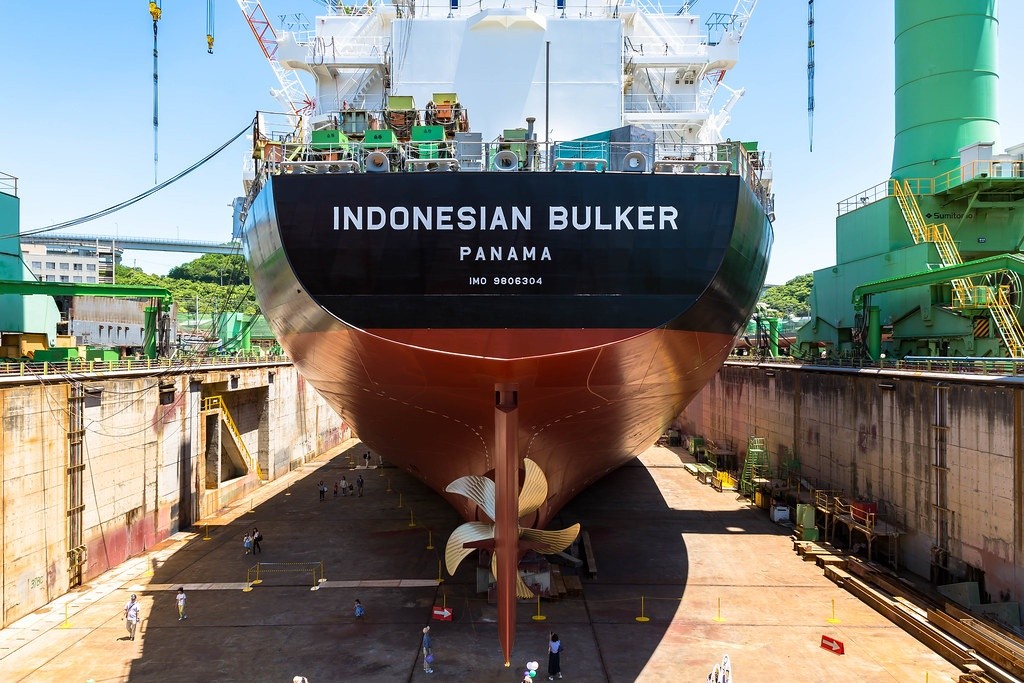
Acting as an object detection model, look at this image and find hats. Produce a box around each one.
[527,661,539,670]
[524,676,532,683]
[422,626,430,634]
[529,670,536,677]
[131,594,136,599]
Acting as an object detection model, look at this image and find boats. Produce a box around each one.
[150,0,814,666]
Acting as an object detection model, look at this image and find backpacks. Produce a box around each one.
[363,453,368,459]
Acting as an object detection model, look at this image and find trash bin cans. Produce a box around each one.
[753,477,770,508]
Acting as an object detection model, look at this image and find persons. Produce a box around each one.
[357,474,364,497]
[347,483,354,496]
[423,625,434,673]
[333,480,338,497]
[353,599,365,616]
[121,594,140,641]
[363,451,371,468]
[252,527,263,554]
[243,531,253,554]
[339,475,349,496]
[175,588,187,621]
[547,633,563,681]
[317,480,328,501]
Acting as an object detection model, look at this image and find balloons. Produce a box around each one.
[523,660,539,678]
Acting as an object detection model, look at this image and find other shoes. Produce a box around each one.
[424,668,433,673]
[245,549,251,554]
[184,615,187,618]
[549,677,553,680]
[179,617,183,620]
[129,637,134,641]
[259,549,261,552]
[253,550,255,554]
[557,675,562,679]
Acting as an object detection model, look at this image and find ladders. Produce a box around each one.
[740,437,774,496]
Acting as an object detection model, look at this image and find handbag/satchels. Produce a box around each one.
[126,612,128,618]
[426,654,434,664]
[255,536,263,541]
[558,645,564,652]
[324,486,328,492]
